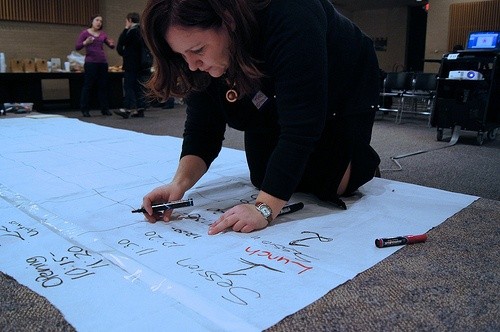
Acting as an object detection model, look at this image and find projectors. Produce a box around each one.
[449,70,483,80]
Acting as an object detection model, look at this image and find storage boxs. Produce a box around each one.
[9,58,48,73]
[3,103,34,113]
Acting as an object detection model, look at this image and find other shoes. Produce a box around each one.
[101,109,112,115]
[83,112,91,116]
[131,108,145,117]
[114,110,131,119]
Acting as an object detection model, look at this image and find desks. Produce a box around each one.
[0,72,162,115]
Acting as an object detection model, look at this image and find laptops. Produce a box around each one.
[457,31,500,52]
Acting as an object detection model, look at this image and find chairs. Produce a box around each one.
[376,72,438,125]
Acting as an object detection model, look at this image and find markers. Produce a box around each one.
[375,234,427,249]
[131,198,193,213]
[277,202,304,217]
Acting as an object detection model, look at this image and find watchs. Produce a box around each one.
[255,202,274,226]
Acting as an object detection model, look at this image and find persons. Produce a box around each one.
[75,15,115,117]
[113,12,153,119]
[142,0,381,234]
